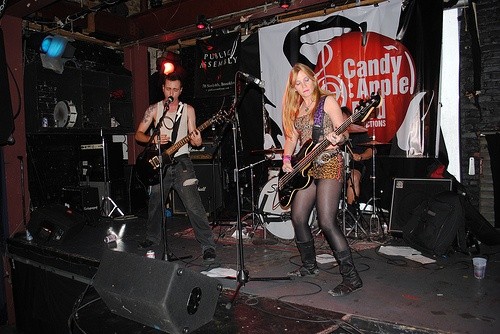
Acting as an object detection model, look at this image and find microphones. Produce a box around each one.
[164,96,174,108]
[240,72,265,88]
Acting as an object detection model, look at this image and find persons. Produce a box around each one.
[282,63,363,296]
[134,73,216,262]
[340,107,377,221]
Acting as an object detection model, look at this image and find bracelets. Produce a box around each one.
[360,154,362,160]
[282,155,292,160]
[338,135,347,145]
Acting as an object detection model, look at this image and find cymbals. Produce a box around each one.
[346,123,368,133]
[251,149,285,155]
[356,140,394,146]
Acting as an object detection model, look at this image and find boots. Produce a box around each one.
[346,203,359,232]
[288,237,320,280]
[327,247,364,298]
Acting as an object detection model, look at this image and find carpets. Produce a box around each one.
[173,219,392,255]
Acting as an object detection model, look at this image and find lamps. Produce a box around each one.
[280,0,291,8]
[195,15,211,30]
[156,58,174,76]
[196,38,212,52]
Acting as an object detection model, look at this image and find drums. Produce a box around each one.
[338,152,351,172]
[54,100,78,128]
[257,175,319,243]
[267,167,281,181]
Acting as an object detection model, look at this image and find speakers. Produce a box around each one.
[25,206,87,246]
[93,250,222,334]
[388,178,456,237]
[171,160,226,215]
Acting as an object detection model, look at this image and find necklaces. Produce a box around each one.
[303,99,315,112]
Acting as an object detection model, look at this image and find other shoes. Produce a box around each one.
[203,248,217,260]
[135,244,153,252]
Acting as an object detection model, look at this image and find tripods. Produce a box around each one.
[314,142,388,243]
[94,129,124,222]
[215,80,295,309]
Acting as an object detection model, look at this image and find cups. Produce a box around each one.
[473,258,487,279]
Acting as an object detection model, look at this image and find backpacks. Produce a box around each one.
[401,193,480,258]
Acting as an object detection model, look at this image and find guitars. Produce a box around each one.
[277,90,383,210]
[134,113,222,184]
